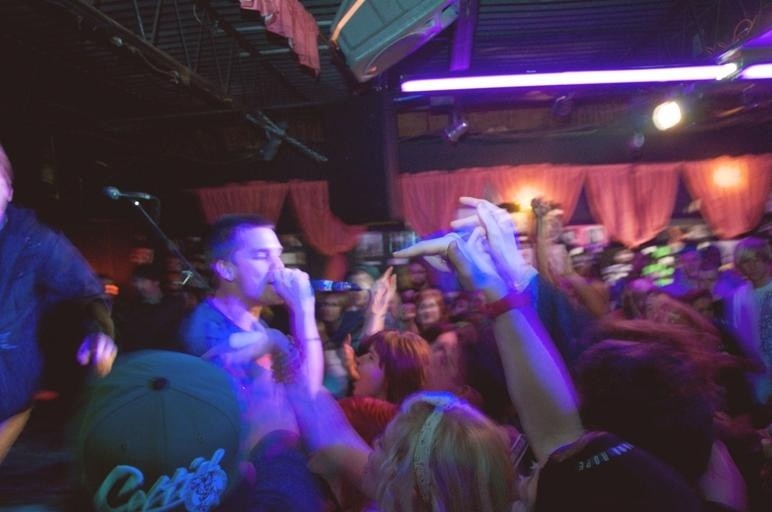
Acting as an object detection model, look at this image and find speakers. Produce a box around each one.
[322,90,403,225]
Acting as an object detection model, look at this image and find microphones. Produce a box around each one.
[102,185,158,201]
[309,278,362,294]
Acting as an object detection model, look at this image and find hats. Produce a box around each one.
[535,426,708,511]
[75,350,249,511]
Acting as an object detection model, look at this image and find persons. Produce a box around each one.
[0,143,771,511]
